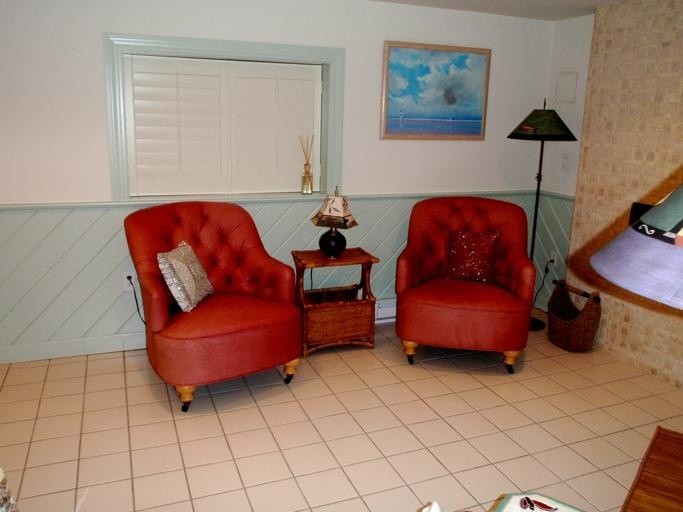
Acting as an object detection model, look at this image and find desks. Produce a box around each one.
[619,424,683,511]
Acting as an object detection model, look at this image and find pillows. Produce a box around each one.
[157,240,214,313]
[447,228,503,283]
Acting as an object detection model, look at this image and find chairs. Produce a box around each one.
[396,197,537,374]
[124,201,303,412]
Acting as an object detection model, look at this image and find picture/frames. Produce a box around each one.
[380,39,492,141]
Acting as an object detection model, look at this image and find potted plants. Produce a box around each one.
[298,134,316,195]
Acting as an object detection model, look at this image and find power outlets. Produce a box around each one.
[123,272,135,291]
[549,250,557,268]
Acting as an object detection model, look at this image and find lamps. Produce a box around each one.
[566,161,683,318]
[310,185,358,259]
[506,98,577,331]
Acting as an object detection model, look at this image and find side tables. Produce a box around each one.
[290,246,381,358]
